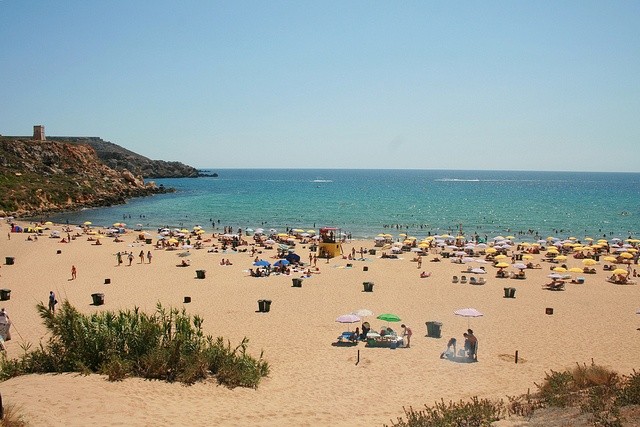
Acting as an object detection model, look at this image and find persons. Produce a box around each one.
[47,291,58,314]
[139,249,145,263]
[335,313,360,330]
[399,323,413,348]
[466,328,478,361]
[147,250,153,263]
[70,263,77,279]
[463,332,474,361]
[116,251,123,265]
[127,251,134,265]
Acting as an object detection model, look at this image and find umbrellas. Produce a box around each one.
[401,228,525,271]
[352,308,374,327]
[453,306,484,328]
[375,312,401,327]
[526,232,640,291]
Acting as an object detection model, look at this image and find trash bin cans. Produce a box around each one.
[363,282,374,291]
[510,288,516,297]
[504,287,509,297]
[0,289,11,300]
[91,293,104,304]
[426,321,442,338]
[257,299,271,311]
[442,252,449,258]
[291,279,301,287]
[6,257,15,264]
[370,249,376,255]
[592,255,595,259]
[146,239,152,244]
[596,255,599,261]
[515,254,524,260]
[196,270,205,278]
